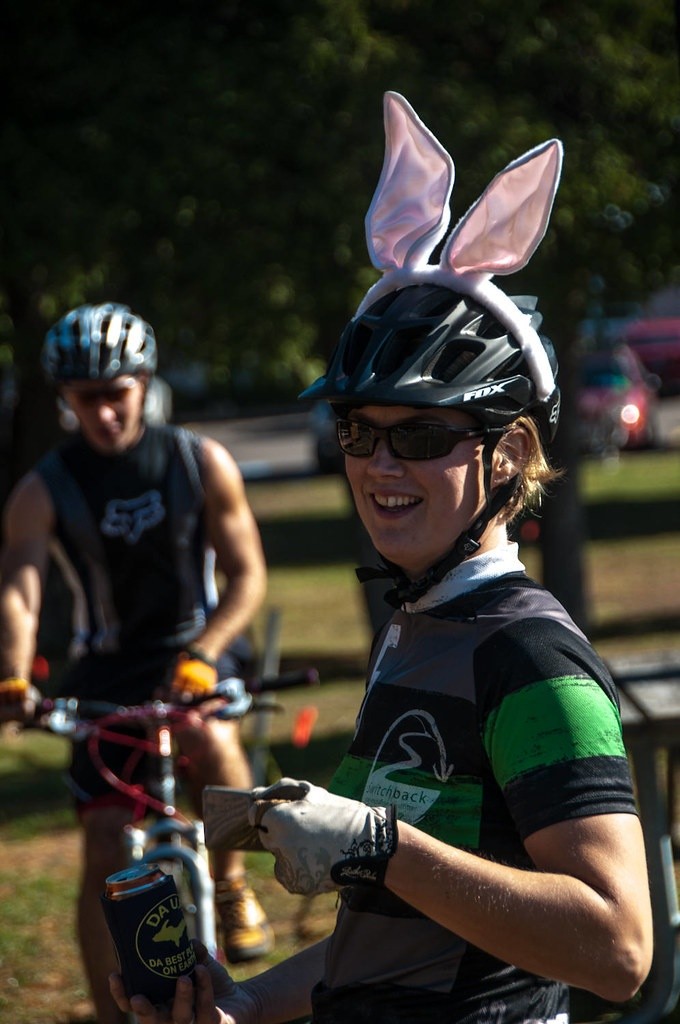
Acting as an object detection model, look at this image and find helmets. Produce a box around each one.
[299,282,558,415]
[39,301,158,380]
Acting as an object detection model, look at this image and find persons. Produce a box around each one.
[0,301,273,1024]
[108,283,654,1024]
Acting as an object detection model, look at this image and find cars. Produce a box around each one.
[555,346,665,450]
[613,291,680,389]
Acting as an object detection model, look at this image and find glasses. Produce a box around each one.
[72,377,138,404]
[334,415,507,460]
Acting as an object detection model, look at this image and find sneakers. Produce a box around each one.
[214,875,276,963]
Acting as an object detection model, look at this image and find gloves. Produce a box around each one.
[245,776,399,897]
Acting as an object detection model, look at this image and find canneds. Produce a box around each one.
[103,862,169,902]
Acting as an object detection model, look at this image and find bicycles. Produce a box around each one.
[0,666,324,975]
